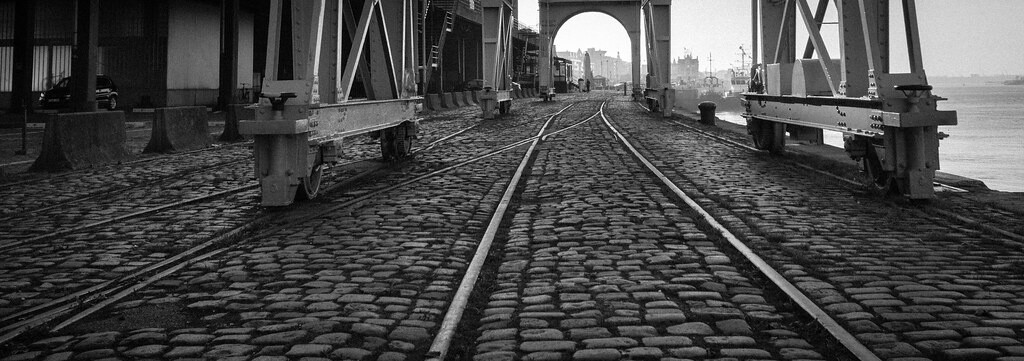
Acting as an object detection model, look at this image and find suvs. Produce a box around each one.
[39,73,120,110]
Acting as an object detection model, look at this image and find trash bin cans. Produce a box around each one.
[698,102,716,125]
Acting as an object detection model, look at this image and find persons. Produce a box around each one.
[587,79,590,91]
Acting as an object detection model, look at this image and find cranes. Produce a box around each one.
[739,46,753,72]
